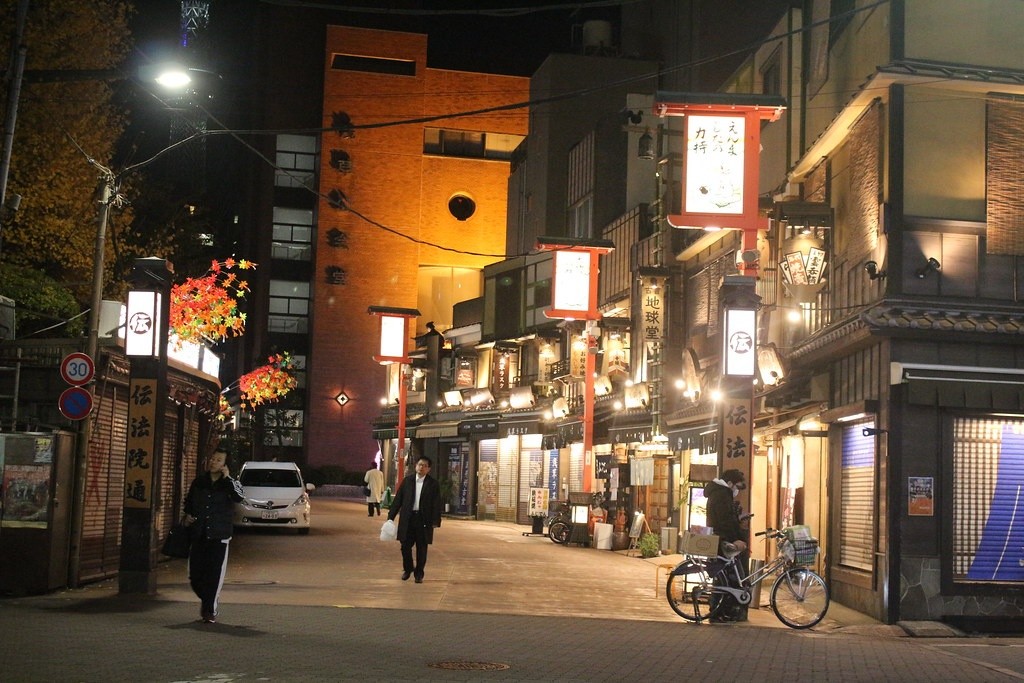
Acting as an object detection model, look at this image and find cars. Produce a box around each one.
[233,460,316,535]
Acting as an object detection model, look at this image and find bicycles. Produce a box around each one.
[665,527,832,630]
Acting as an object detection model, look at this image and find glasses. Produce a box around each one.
[734,483,746,490]
[417,464,429,469]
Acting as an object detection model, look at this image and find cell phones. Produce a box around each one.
[224,459,229,467]
[741,514,754,521]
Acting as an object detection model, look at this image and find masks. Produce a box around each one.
[732,487,739,498]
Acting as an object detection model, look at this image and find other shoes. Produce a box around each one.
[368,514,374,517]
[413,571,422,583]
[402,567,414,580]
[378,511,381,516]
[201,608,219,623]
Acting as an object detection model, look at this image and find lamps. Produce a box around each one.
[863,428,889,437]
[380,336,783,421]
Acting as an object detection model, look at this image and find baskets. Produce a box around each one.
[789,539,818,566]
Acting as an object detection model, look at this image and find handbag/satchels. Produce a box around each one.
[363,487,371,496]
[161,515,191,559]
[380,519,396,541]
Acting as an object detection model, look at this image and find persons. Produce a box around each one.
[704,469,748,626]
[387,456,441,584]
[364,462,384,518]
[183,449,246,621]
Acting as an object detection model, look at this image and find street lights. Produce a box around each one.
[0,40,198,214]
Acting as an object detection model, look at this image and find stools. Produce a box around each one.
[656,564,681,599]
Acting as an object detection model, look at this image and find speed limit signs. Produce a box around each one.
[60,351,95,387]
